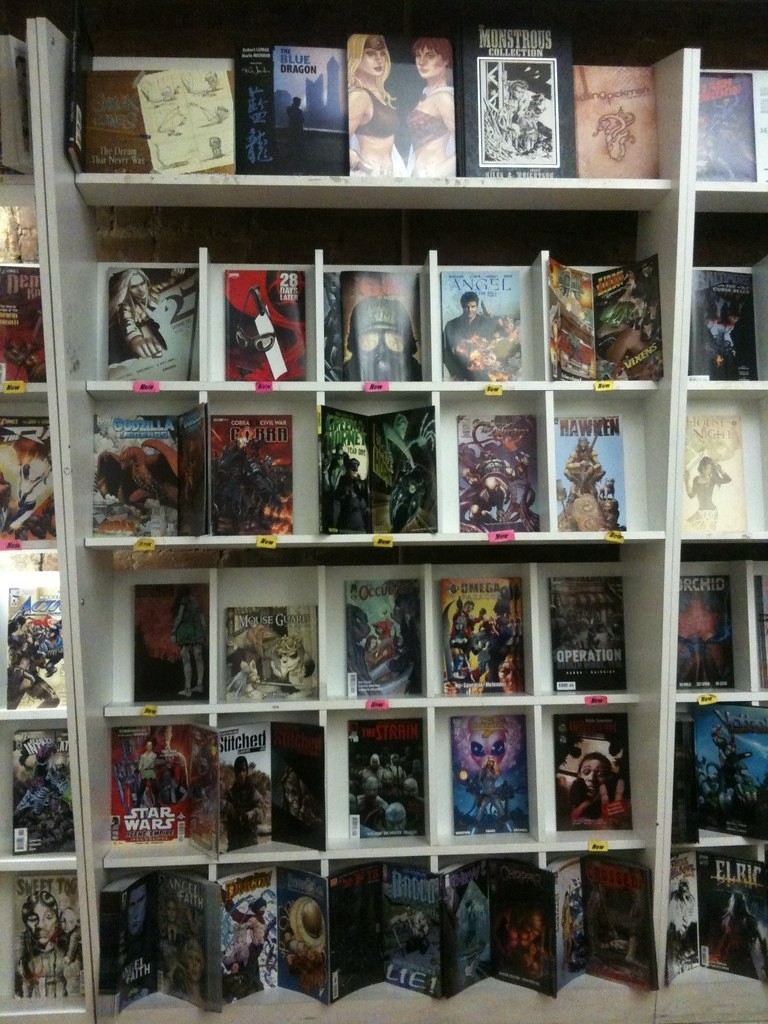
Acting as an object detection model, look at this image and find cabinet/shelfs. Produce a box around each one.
[0,18,768,1024]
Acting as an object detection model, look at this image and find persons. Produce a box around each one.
[160,899,186,949]
[442,292,506,381]
[478,612,515,690]
[218,756,267,849]
[560,752,625,828]
[451,601,484,667]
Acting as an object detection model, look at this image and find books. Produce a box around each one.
[6,569,768,706]
[11,849,768,1013]
[0,402,745,538]
[0,13,759,182]
[0,253,760,381]
[13,704,768,843]
